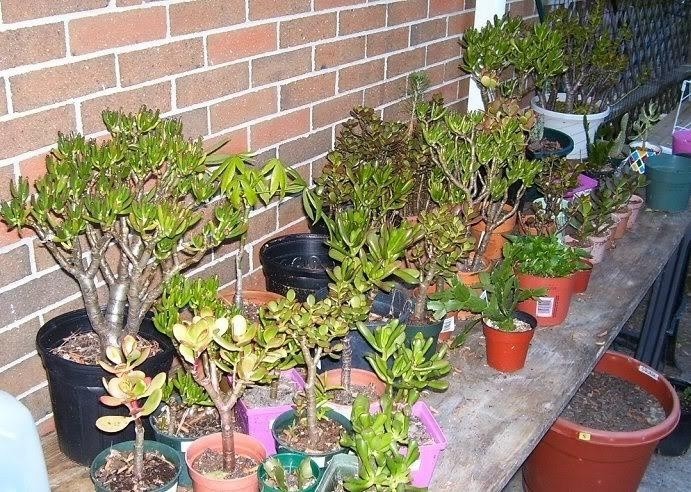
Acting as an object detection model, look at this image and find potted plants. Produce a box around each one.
[655,375,691,456]
